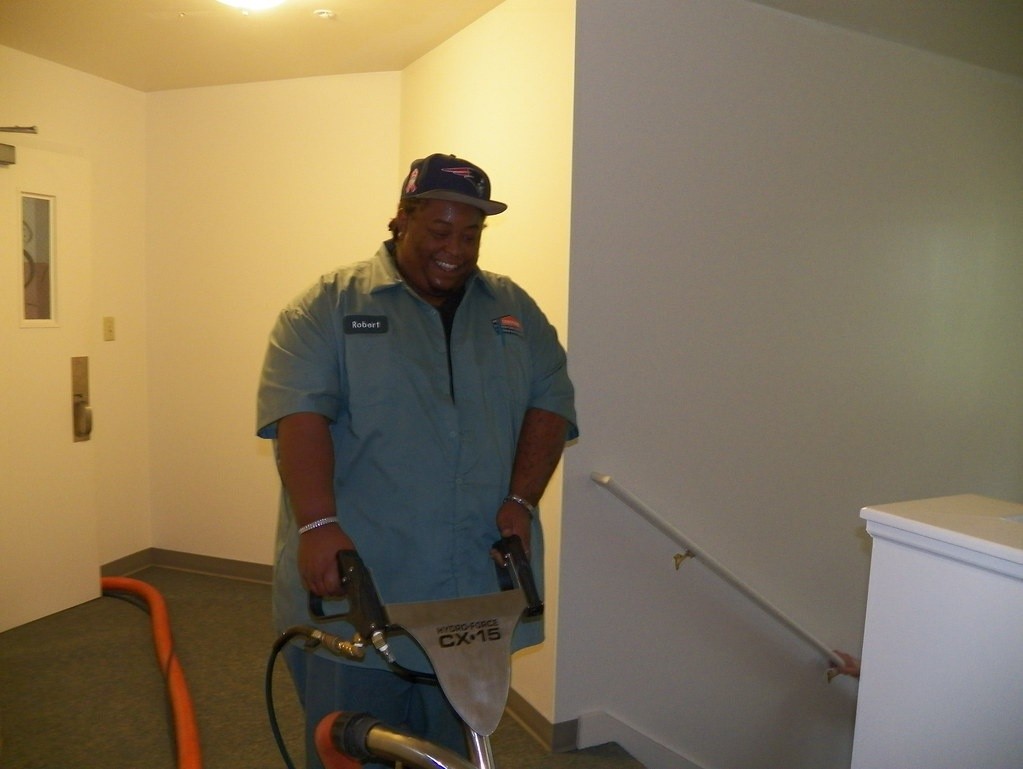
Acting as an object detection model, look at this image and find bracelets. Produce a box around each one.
[503,495,535,520]
[298,516,337,537]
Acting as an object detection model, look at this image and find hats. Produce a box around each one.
[400,154,507,215]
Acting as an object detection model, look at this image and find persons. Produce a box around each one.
[256,154,579,769]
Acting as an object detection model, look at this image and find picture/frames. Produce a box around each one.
[20,191,60,329]
